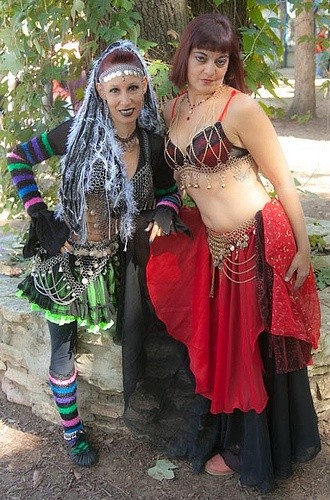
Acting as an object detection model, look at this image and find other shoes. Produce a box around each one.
[204,444,242,476]
[63,418,100,468]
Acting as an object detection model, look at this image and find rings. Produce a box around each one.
[162,230,169,235]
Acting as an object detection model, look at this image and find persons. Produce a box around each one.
[154,13,322,490]
[5,41,188,467]
[315,23,329,77]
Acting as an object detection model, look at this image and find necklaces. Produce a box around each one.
[183,86,223,122]
[111,127,143,153]
